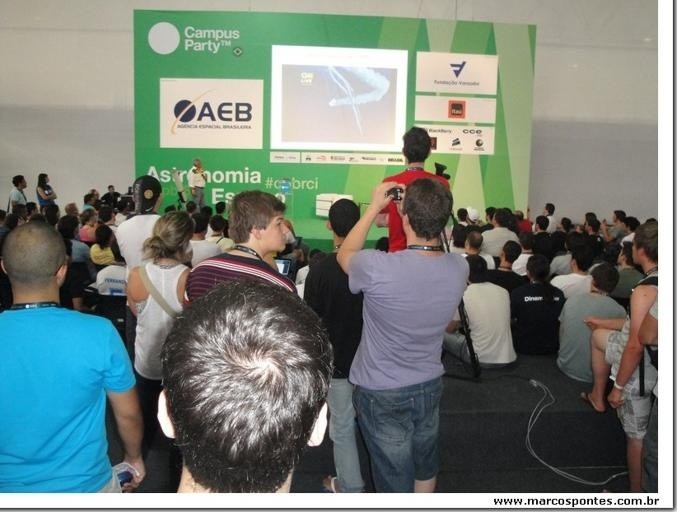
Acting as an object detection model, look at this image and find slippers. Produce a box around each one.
[323,475,340,493]
[580,391,606,414]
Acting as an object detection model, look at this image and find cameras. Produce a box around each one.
[273,256,292,276]
[386,187,404,200]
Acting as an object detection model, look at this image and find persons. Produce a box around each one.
[602,210,627,242]
[457,208,469,227]
[90,225,114,265]
[200,205,213,240]
[545,231,589,280]
[305,199,368,493]
[37,173,58,214]
[101,185,131,206]
[79,209,95,242]
[65,202,77,217]
[211,202,231,237]
[443,254,517,369]
[513,210,532,232]
[581,218,658,493]
[556,263,627,383]
[481,207,496,233]
[0,208,5,228]
[554,217,572,244]
[551,231,567,254]
[295,249,325,298]
[576,212,598,233]
[116,201,126,225]
[116,175,162,364]
[480,208,520,258]
[586,219,602,245]
[8,175,28,207]
[126,211,192,470]
[97,236,127,295]
[190,212,222,267]
[532,232,553,261]
[483,241,523,288]
[184,189,299,303]
[1,222,146,493]
[206,215,235,252]
[90,189,102,208]
[509,255,566,355]
[542,203,562,233]
[550,243,593,299]
[59,215,95,285]
[157,276,334,493]
[454,208,480,247]
[187,201,197,212]
[96,205,118,235]
[374,127,451,253]
[619,217,643,247]
[533,216,552,236]
[12,204,27,225]
[40,203,60,229]
[460,231,496,270]
[607,242,646,299]
[337,177,470,492]
[0,213,18,238]
[26,202,38,220]
[512,230,534,276]
[82,194,96,214]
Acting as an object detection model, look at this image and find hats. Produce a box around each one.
[132,174,162,214]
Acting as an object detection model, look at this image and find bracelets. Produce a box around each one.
[614,380,624,390]
